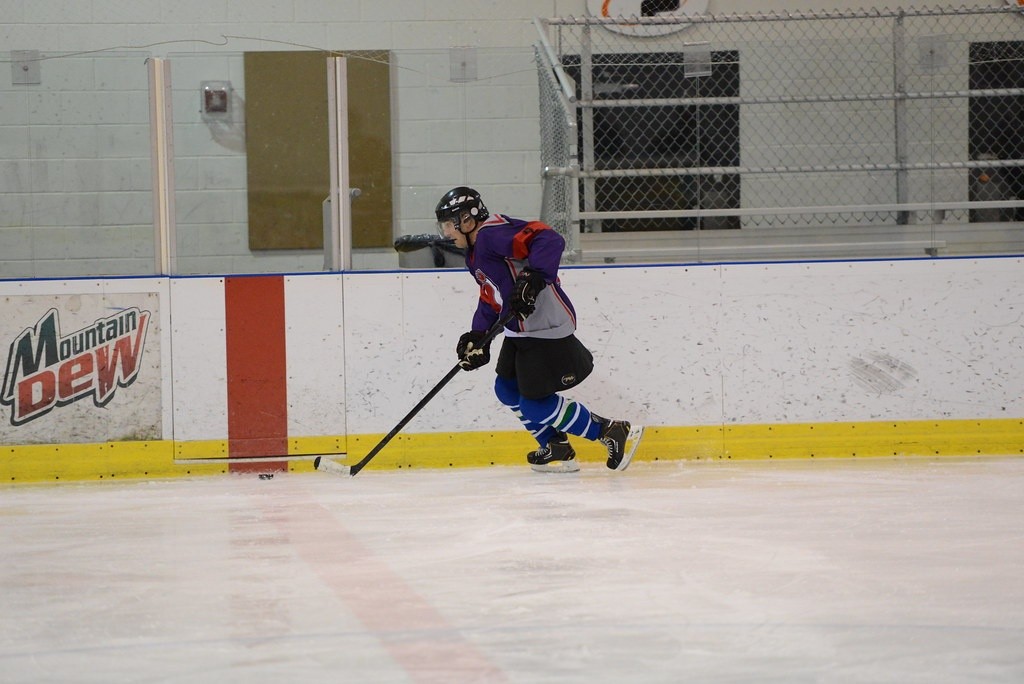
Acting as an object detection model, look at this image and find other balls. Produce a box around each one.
[258,473,274,480]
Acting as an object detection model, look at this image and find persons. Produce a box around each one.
[435,186,644,472]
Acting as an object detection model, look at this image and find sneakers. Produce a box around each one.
[527,430,580,472]
[590,411,644,471]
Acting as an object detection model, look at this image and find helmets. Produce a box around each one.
[435,187,489,228]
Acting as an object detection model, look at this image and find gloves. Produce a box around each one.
[457,329,490,371]
[508,265,546,321]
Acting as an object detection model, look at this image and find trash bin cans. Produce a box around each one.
[438,239,467,268]
[396,235,436,268]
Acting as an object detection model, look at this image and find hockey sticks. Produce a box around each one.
[313,312,515,478]
